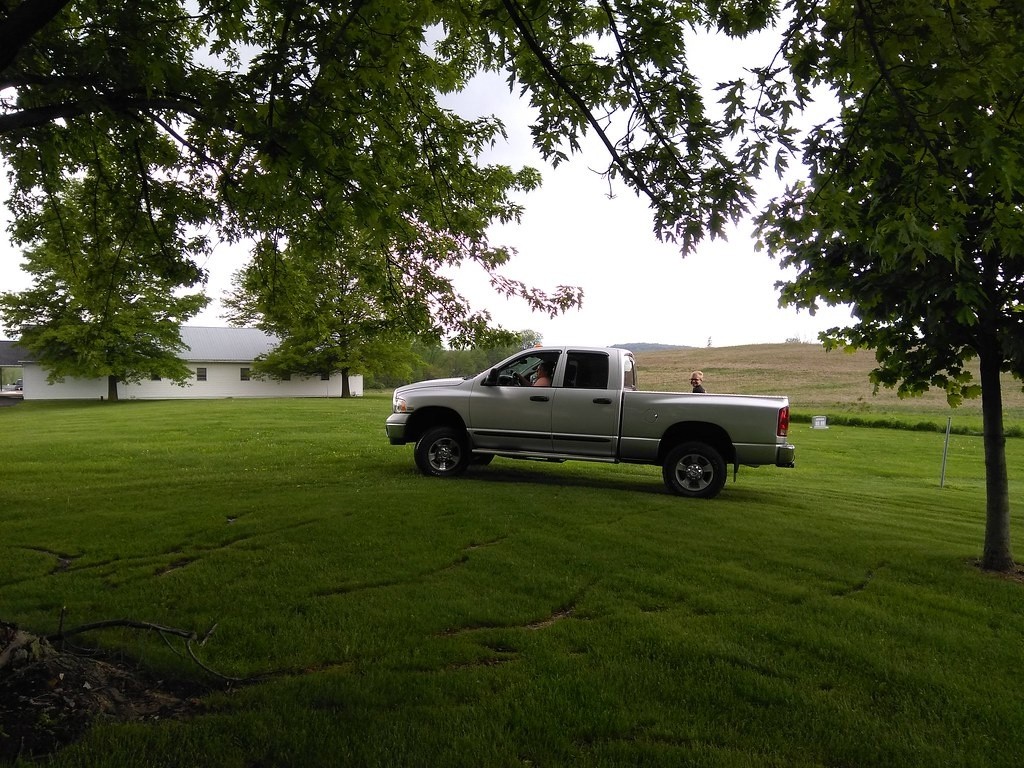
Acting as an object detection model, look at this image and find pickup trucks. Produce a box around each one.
[385,345,796,499]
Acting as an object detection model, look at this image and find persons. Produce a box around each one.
[690,371,707,394]
[519,363,551,387]
